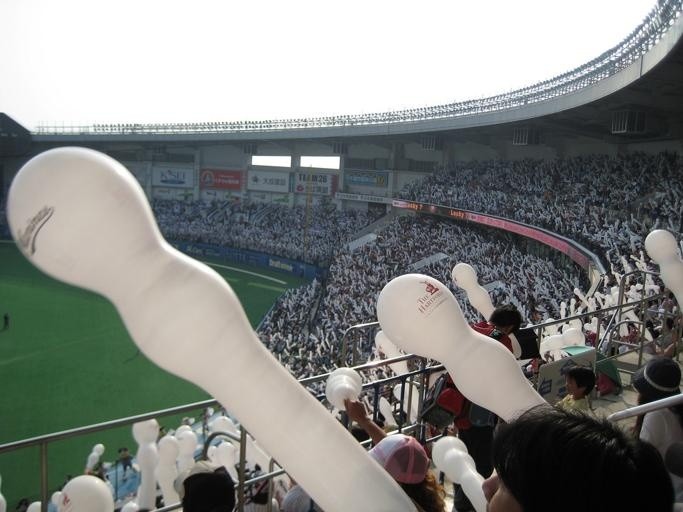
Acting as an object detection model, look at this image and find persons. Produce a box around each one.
[0,148,683,512]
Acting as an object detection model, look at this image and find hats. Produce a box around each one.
[367,434,429,484]
[631,357,681,400]
